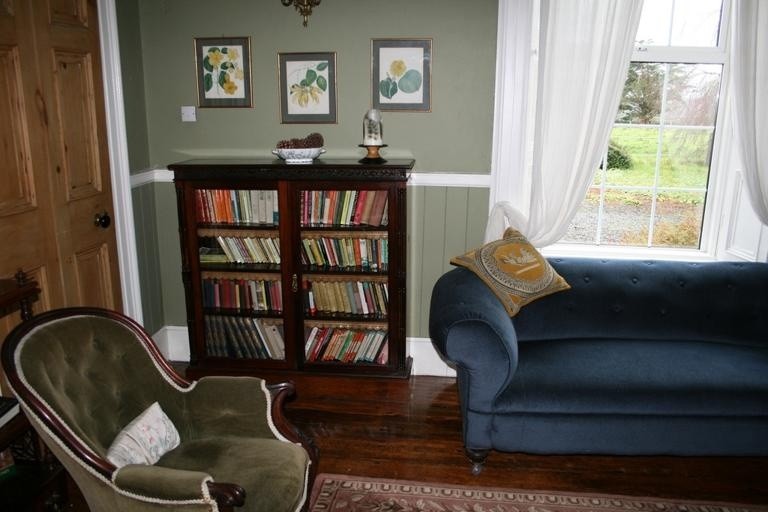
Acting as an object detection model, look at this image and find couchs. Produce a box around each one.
[425,255,765,478]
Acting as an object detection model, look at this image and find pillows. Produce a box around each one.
[449,223,576,318]
[97,402,183,473]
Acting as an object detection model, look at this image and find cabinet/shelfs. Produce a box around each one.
[165,157,419,382]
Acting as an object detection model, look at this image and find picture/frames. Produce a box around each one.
[192,35,255,110]
[275,49,340,125]
[369,35,433,115]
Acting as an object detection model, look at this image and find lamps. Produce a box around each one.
[281,0,322,28]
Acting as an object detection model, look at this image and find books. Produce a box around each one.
[189,184,386,363]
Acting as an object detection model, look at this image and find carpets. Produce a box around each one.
[299,472,766,509]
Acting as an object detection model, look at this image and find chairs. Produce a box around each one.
[2,305,322,512]
